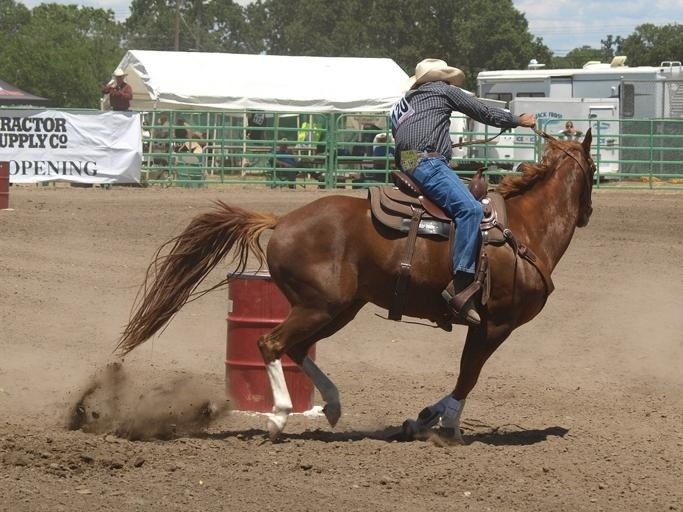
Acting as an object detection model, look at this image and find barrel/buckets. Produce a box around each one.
[0,159,10,211]
[223,271,316,413]
[176,160,204,187]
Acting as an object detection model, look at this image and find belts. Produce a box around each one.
[415,152,439,159]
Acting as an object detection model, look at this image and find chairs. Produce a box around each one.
[262,171,305,189]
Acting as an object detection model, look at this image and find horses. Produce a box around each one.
[109,126,598,444]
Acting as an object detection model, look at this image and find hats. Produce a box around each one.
[406,57,466,91]
[111,69,127,78]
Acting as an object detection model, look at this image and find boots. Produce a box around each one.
[441,271,482,325]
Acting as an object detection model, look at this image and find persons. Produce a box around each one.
[393,55,534,327]
[268,137,320,188]
[246,111,264,143]
[154,115,170,140]
[557,122,582,141]
[173,117,192,138]
[102,67,132,109]
[142,121,151,168]
